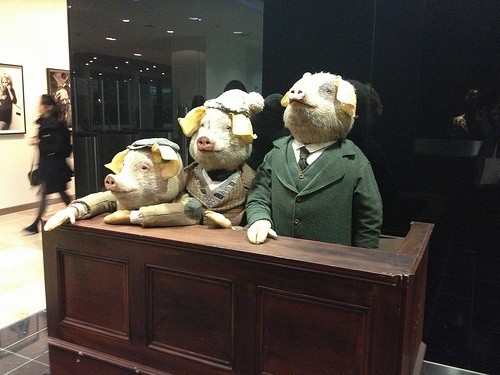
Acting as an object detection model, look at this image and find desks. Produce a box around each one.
[40,210,434,375]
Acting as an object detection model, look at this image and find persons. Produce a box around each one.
[52,87,72,128]
[23,94,73,232]
[0,73,17,131]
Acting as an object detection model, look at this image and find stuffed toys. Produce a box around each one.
[177,89,266,228]
[43,137,201,232]
[245,71,383,248]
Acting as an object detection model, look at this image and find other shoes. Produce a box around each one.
[25,225,39,233]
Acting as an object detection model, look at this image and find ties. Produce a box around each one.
[299,148,308,172]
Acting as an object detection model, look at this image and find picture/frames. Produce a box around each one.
[46,67,72,128]
[0,63,28,135]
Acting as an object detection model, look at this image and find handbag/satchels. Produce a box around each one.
[28,169,42,186]
